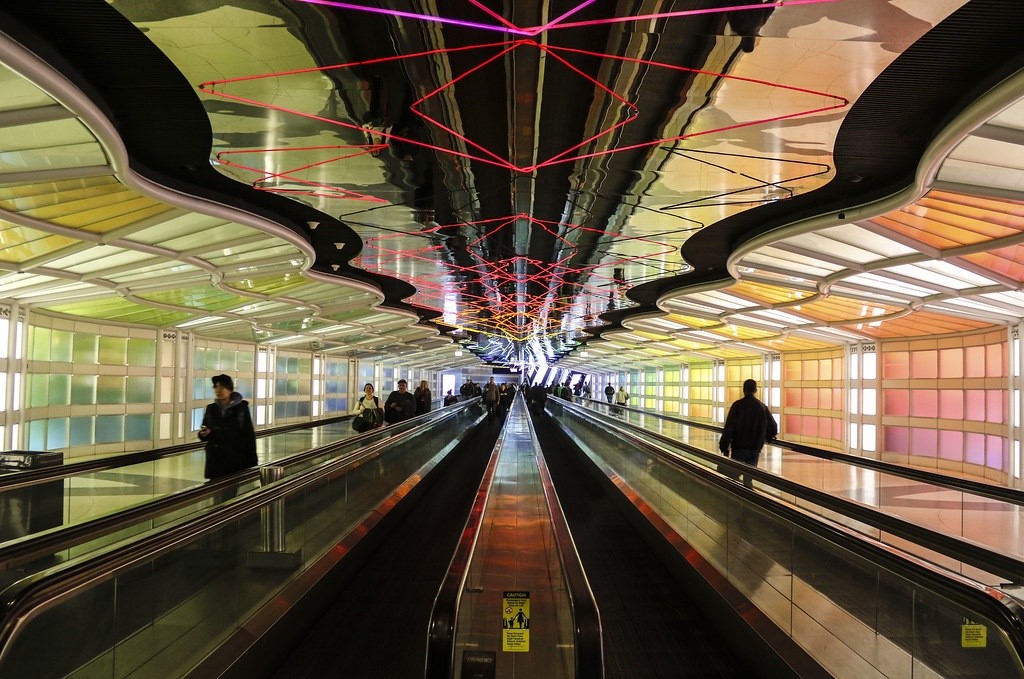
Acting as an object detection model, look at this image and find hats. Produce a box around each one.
[397,379,406,383]
[212,374,234,391]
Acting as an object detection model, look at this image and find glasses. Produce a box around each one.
[212,384,226,389]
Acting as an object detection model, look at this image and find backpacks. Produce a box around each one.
[481,384,490,400]
[564,387,571,399]
[460,385,465,394]
[383,391,395,423]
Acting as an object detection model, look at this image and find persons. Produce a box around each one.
[617,386,627,406]
[444,389,458,406]
[498,383,510,415]
[483,377,500,426]
[353,384,385,447]
[560,382,572,402]
[717,378,777,491]
[385,378,415,438]
[461,379,482,404]
[605,383,615,403]
[413,381,432,423]
[503,380,560,418]
[580,380,592,398]
[198,374,258,505]
[573,383,581,396]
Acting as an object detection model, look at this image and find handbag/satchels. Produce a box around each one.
[715,463,741,481]
[352,413,369,432]
[586,386,591,393]
[625,393,630,400]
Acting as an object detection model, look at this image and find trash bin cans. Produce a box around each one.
[0,447,67,545]
